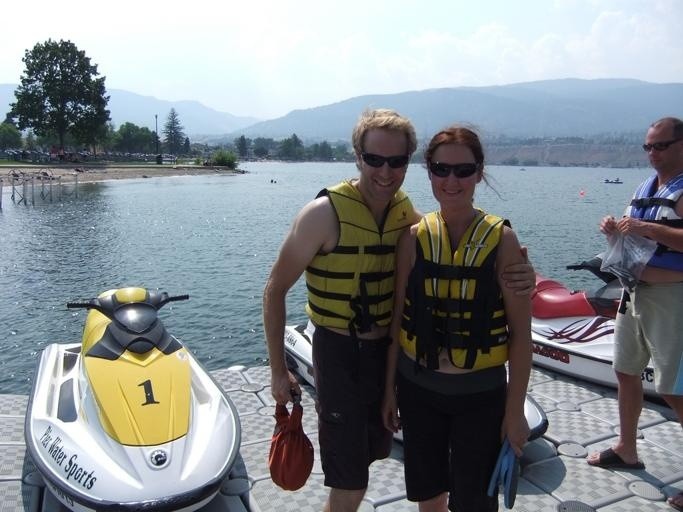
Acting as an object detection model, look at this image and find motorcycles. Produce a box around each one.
[285,318,548,440]
[27,289,242,512]
[530,251,663,396]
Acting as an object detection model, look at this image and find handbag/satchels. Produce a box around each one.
[269,393,313,490]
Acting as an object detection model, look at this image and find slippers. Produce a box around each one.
[487,437,518,509]
[589,448,643,469]
[666,490,683,512]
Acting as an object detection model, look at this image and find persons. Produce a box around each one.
[382,121,534,512]
[263,105,538,512]
[586,116,683,512]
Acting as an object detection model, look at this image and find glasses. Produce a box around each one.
[428,160,479,178]
[642,138,681,151]
[360,151,410,168]
